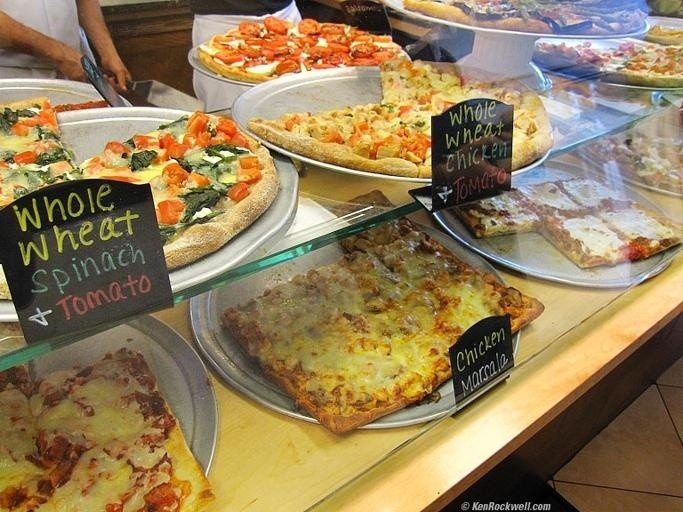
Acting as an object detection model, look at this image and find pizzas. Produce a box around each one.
[219,190,545,436]
[533,25,683,194]
[0,322,217,512]
[198,15,406,84]
[403,0,648,35]
[0,96,279,300]
[451,172,683,268]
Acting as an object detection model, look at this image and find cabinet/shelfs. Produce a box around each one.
[1,0,683,511]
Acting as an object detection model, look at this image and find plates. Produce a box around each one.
[184,25,414,87]
[528,10,683,93]
[1,314,220,482]
[1,75,134,110]
[1,105,300,322]
[430,159,682,290]
[186,214,526,434]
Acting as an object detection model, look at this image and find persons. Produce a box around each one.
[0,1,134,94]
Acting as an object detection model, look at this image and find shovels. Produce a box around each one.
[94,64,205,117]
